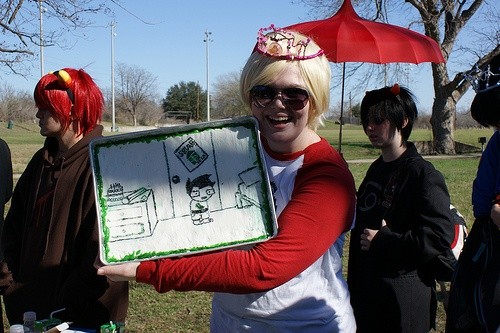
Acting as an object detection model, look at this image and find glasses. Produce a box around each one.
[248,84,312,111]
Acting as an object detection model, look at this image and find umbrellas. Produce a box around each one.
[274,1,446,152]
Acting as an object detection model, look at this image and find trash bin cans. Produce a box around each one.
[8,120,13,129]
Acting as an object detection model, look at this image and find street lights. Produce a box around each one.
[109,21,117,133]
[405,62,412,90]
[203,28,214,123]
[39,1,47,77]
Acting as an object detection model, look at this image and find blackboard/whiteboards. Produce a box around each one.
[88,115,280,264]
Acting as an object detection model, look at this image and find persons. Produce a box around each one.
[93,29,355,333]
[346,85,452,333]
[0,68,130,333]
[446,57,500,333]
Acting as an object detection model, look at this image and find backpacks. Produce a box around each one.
[402,161,469,281]
